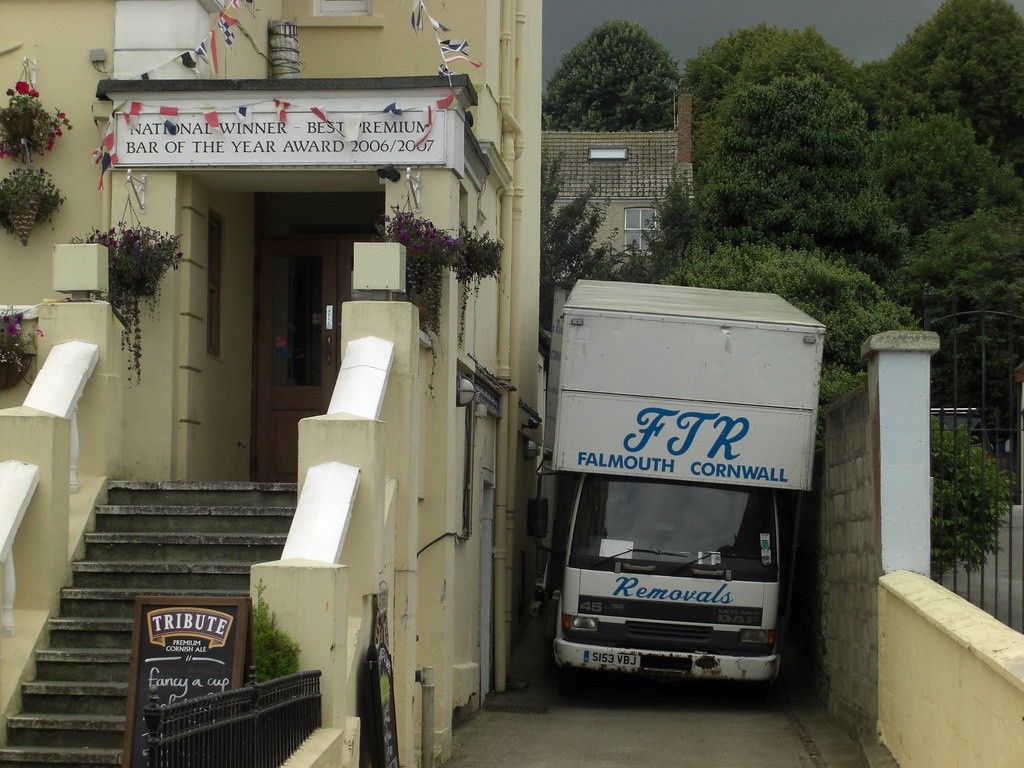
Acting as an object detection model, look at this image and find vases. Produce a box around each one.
[120,272,153,296]
[0,367,19,390]
[9,111,33,138]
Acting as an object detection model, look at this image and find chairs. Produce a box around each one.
[679,488,736,531]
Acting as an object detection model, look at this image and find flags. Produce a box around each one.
[94,0,470,192]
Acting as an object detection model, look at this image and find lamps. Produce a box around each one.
[524,439,541,460]
[457,375,478,407]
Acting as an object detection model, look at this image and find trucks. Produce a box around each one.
[526,279,829,698]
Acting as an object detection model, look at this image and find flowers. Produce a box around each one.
[71,222,184,389]
[374,204,458,332]
[0,306,43,375]
[0,168,70,227]
[0,81,71,163]
